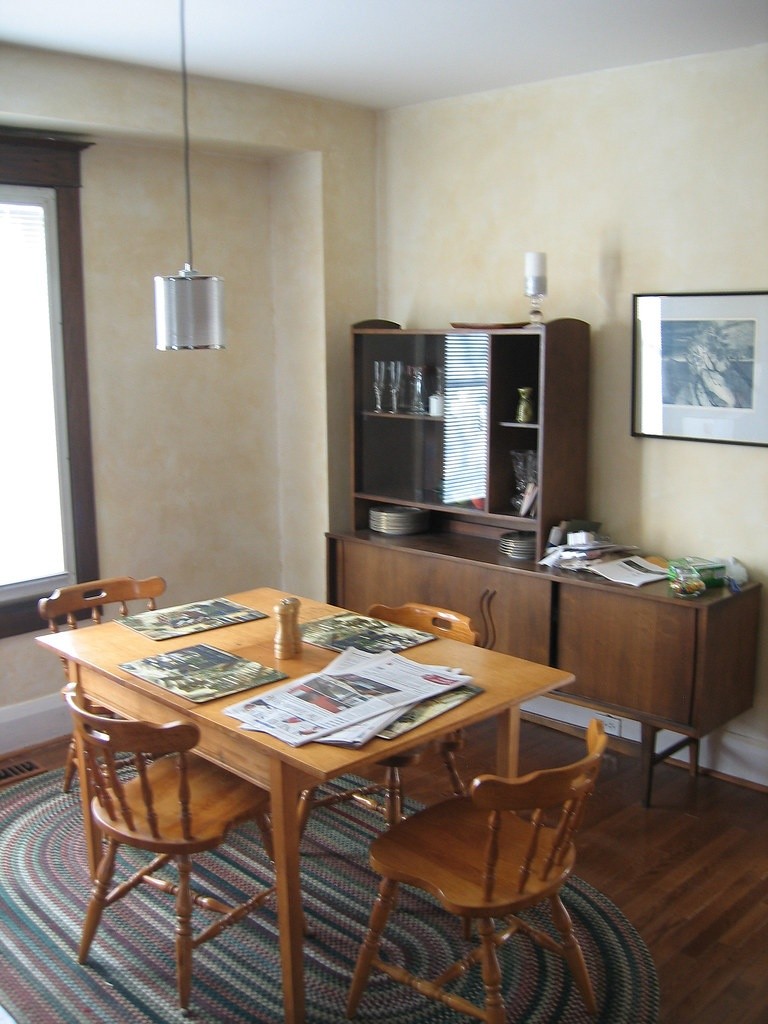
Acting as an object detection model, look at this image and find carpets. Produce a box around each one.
[0,753,659,1024]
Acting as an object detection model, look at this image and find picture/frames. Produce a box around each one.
[629,291,768,447]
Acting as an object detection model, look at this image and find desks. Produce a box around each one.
[34,587,577,1024]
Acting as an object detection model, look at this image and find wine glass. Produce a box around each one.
[372,359,387,413]
[388,360,403,414]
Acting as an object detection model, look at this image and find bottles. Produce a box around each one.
[273,597,302,660]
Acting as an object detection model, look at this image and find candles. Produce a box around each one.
[523,251,547,296]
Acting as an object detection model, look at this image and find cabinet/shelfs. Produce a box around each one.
[325,319,761,808]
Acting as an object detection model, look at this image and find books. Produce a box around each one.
[586,555,671,586]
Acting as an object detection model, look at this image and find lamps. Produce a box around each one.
[153,0,224,352]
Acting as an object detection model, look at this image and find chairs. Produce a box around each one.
[344,717,609,1024]
[61,683,309,1017]
[37,576,168,793]
[298,603,481,847]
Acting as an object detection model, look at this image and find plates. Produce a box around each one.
[368,505,431,536]
[497,531,538,561]
